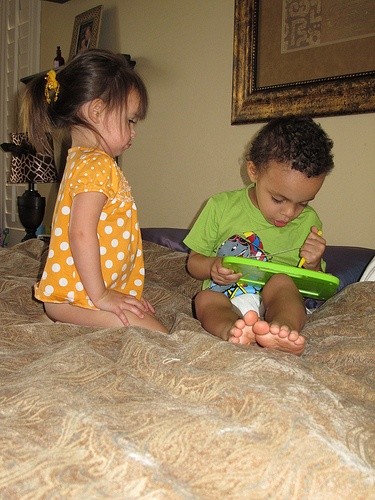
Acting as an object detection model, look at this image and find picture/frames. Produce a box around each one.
[68,5,103,62]
[231,0,375,125]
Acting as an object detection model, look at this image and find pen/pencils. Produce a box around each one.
[298,231,322,267]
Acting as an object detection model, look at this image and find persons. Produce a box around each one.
[184,112,335,356]
[17,48,168,333]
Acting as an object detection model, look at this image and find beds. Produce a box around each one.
[0,227,375,500]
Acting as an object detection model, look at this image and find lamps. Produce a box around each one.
[7,132,60,242]
